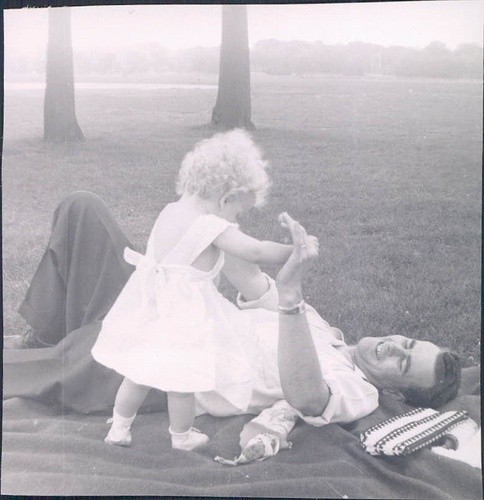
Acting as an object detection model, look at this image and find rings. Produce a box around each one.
[301,243,307,249]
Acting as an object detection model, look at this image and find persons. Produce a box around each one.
[89,125,320,453]
[0,191,462,430]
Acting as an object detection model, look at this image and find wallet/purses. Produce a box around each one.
[360,402,470,457]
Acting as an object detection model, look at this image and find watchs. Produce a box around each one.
[279,298,308,316]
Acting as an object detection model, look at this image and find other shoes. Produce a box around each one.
[3,329,55,352]
[103,410,137,446]
[170,425,208,451]
